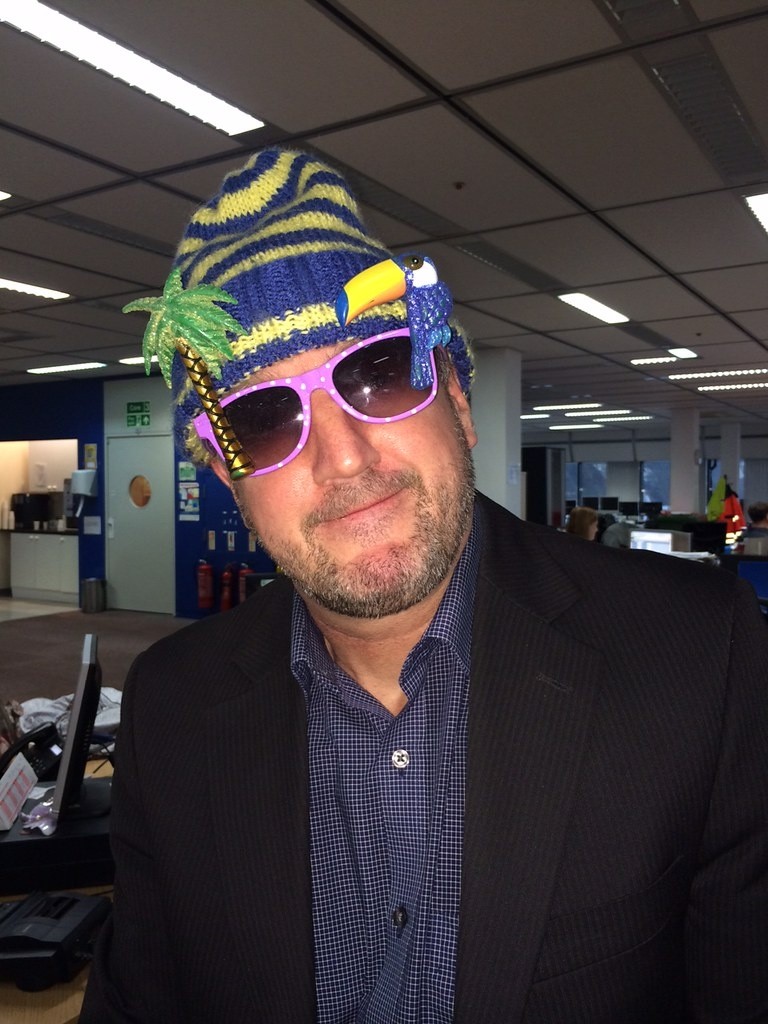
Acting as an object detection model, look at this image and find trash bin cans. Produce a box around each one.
[80,577,107,613]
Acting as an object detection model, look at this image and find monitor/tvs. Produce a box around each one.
[564,497,692,556]
[732,557,768,605]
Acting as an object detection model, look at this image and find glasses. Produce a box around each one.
[192,322,439,479]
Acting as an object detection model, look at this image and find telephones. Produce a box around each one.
[0,720,66,783]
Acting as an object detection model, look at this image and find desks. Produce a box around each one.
[0,733,118,1023]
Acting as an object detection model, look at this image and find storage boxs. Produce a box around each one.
[0,776,118,896]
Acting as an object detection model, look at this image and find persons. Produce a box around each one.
[603,523,637,547]
[77,148,768,1024]
[564,508,598,541]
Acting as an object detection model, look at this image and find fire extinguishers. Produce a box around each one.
[238,562,254,604]
[220,563,234,612]
[197,559,213,608]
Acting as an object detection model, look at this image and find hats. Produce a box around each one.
[123,145,477,475]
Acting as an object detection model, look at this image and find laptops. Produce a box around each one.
[50,633,113,826]
